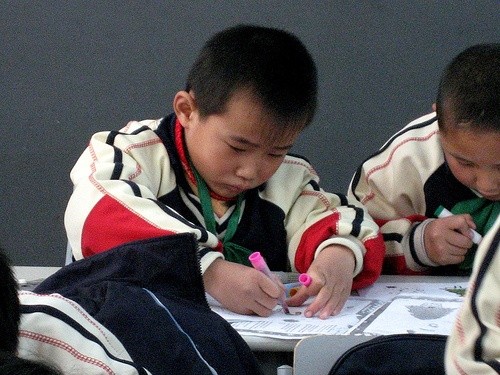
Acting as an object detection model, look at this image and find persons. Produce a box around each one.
[347,43,500,275]
[443,213,500,375]
[0,233,264,375]
[63,24,387,319]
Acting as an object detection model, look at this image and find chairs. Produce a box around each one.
[277,334,374,375]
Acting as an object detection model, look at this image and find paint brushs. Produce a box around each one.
[249,252,290,314]
[298,273,311,286]
[435,206,483,244]
